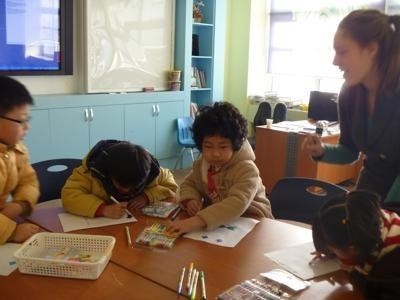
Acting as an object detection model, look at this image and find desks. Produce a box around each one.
[1,200,363,299]
[255,120,364,197]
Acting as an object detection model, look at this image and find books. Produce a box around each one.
[190,103,201,120]
[191,66,206,88]
[142,201,179,219]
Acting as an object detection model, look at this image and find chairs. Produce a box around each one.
[254,102,271,132]
[172,117,198,171]
[28,158,82,204]
[267,176,349,230]
[272,103,287,123]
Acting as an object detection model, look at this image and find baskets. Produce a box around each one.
[14,232,116,280]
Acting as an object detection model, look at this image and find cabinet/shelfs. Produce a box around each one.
[24,91,123,168]
[174,0,223,161]
[123,91,185,163]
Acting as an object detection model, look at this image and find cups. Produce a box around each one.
[317,121,328,132]
[266,119,273,128]
[315,122,323,136]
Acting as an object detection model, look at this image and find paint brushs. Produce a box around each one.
[125,225,132,247]
[171,207,182,220]
[200,197,205,204]
[135,221,177,249]
[190,270,200,300]
[187,268,196,295]
[177,265,186,295]
[110,195,132,216]
[309,254,322,265]
[200,270,207,300]
[187,262,194,287]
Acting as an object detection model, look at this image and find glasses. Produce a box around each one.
[2,116,31,125]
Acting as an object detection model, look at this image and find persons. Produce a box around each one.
[310,189,400,300]
[301,10,400,212]
[61,139,178,220]
[0,75,43,245]
[165,101,275,239]
